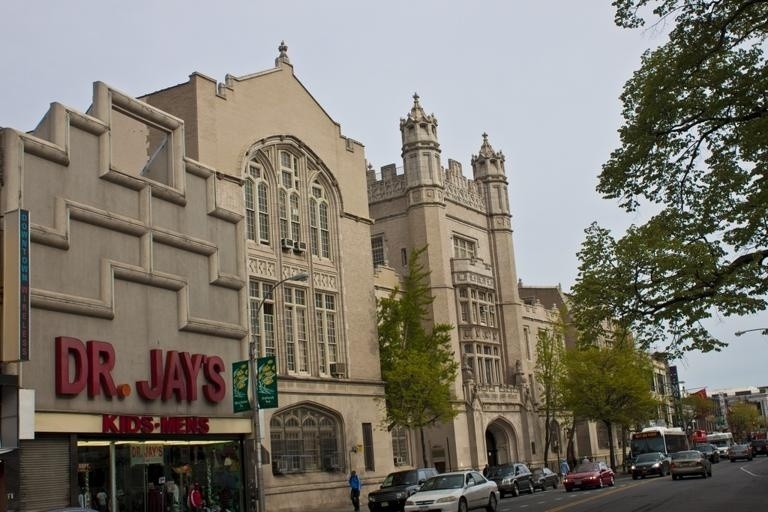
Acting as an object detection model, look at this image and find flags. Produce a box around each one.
[691,387,710,400]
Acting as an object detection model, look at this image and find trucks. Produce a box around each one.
[705,431,735,457]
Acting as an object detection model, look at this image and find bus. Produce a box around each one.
[628,426,690,458]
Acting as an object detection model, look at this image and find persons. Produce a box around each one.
[190,481,202,512]
[78,480,193,512]
[559,458,570,478]
[482,462,489,477]
[348,470,362,512]
[582,455,590,463]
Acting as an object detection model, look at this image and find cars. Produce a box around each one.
[562,461,619,491]
[363,462,557,511]
[625,440,768,477]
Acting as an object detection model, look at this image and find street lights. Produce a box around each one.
[649,381,688,387]
[733,326,768,337]
[249,269,312,512]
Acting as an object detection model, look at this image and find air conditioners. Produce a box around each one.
[281,237,294,249]
[480,305,489,313]
[294,241,307,253]
[329,361,346,376]
